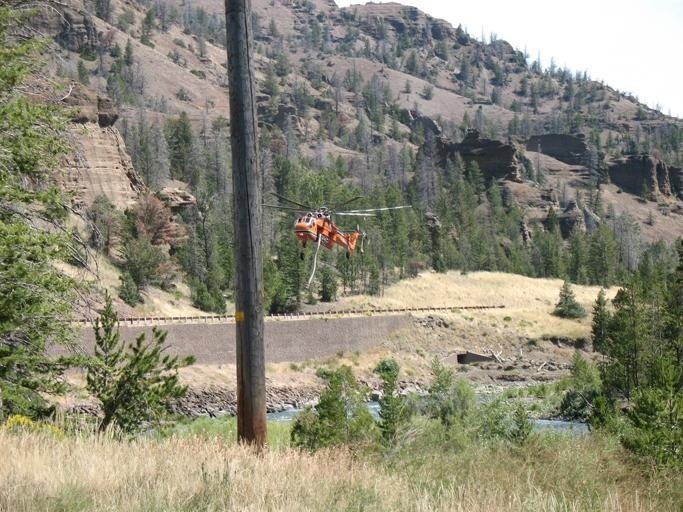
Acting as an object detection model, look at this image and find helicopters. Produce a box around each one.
[260,187,412,259]
